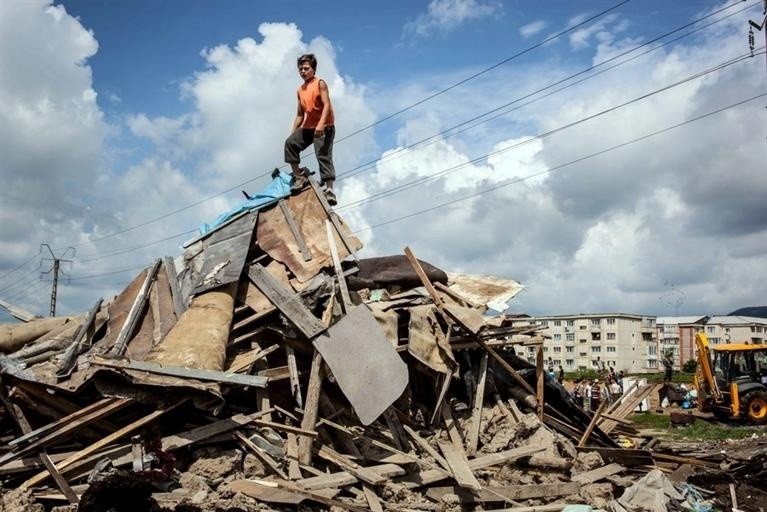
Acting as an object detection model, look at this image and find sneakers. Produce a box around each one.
[324,190,337,205]
[290,175,309,191]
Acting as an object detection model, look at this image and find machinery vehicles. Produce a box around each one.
[694,333,767,425]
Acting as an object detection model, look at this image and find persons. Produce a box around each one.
[558,366,564,384]
[548,369,555,379]
[572,367,624,412]
[284,54,336,204]
[547,357,554,371]
[663,351,675,380]
